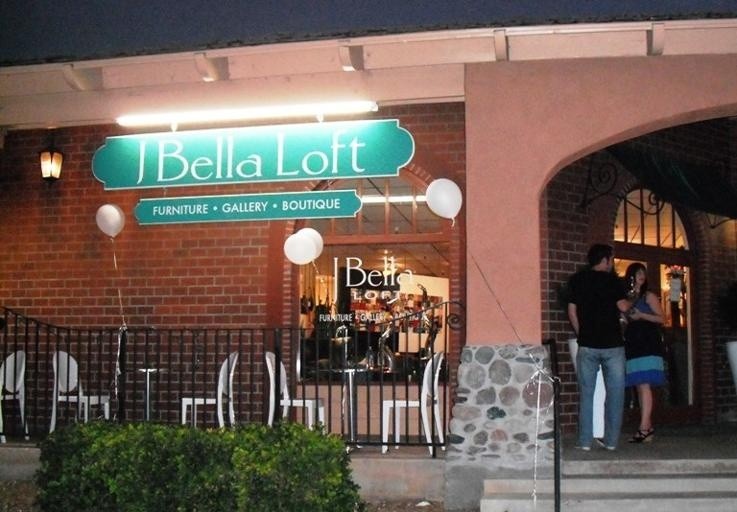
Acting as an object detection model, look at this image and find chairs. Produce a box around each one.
[0,347,111,443]
[260,352,328,443]
[168,348,243,436]
[382,352,450,457]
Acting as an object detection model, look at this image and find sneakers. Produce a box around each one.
[574,439,615,450]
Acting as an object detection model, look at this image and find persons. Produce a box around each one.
[618,263,666,444]
[567,243,640,452]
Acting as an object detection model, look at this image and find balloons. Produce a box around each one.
[295,228,324,259]
[95,204,127,238]
[283,235,317,266]
[426,178,463,226]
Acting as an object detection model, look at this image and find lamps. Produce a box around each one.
[35,130,67,193]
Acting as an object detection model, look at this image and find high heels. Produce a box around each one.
[627,429,654,443]
